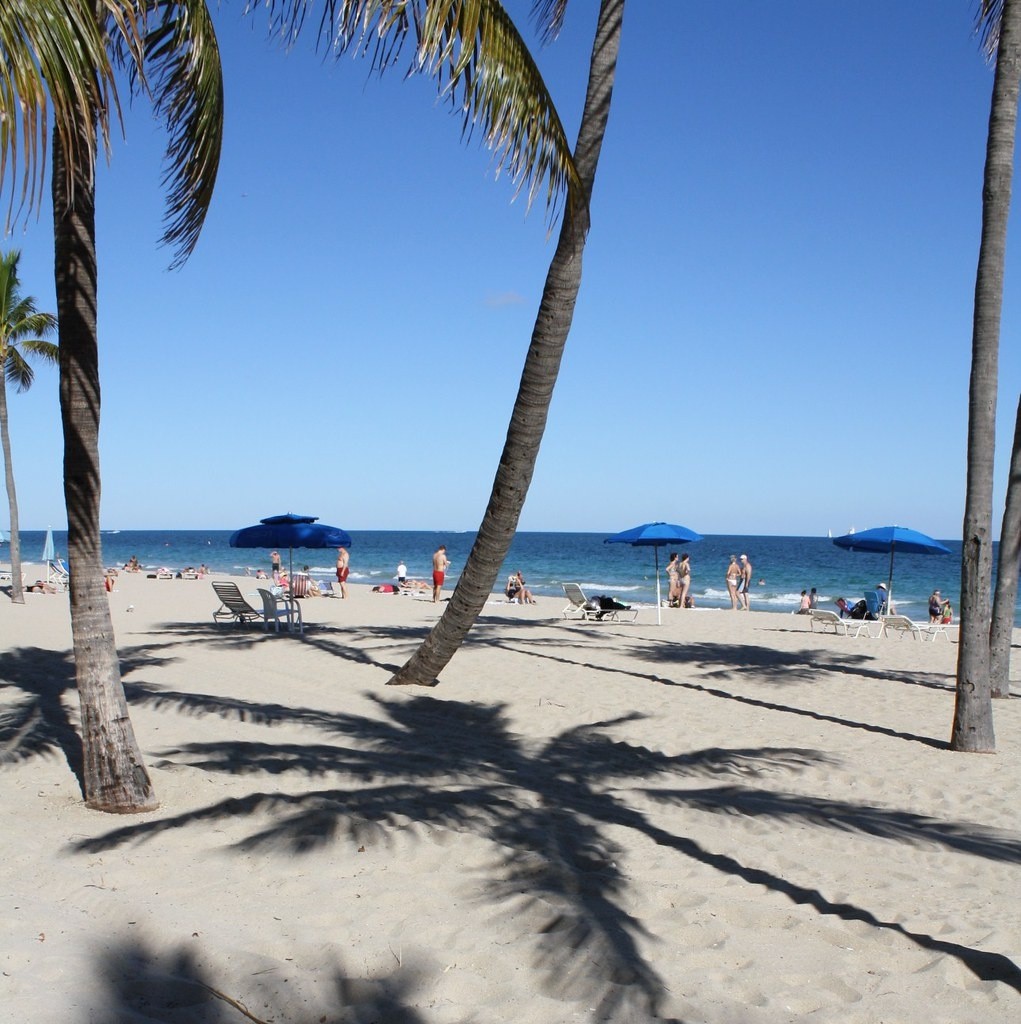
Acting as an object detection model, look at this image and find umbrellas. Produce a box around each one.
[604,521,703,626]
[833,526,952,614]
[228,511,351,630]
[0,529,21,543]
[41,525,54,583]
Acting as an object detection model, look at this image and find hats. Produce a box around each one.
[740,554,748,559]
[877,583,887,591]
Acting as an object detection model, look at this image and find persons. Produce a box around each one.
[337,548,350,599]
[736,555,752,611]
[758,579,764,585]
[726,555,741,610]
[256,569,268,579]
[373,562,430,592]
[506,575,536,605]
[875,583,896,615]
[592,596,631,609]
[270,551,289,590]
[184,564,207,574]
[433,546,450,602]
[303,565,321,596]
[666,553,695,609]
[930,590,953,624]
[124,555,139,572]
[797,588,818,615]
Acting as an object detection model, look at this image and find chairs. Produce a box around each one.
[211,581,299,633]
[270,570,337,600]
[257,588,303,634]
[146,572,199,580]
[49,559,69,586]
[0,571,27,581]
[561,583,639,622]
[808,592,949,642]
